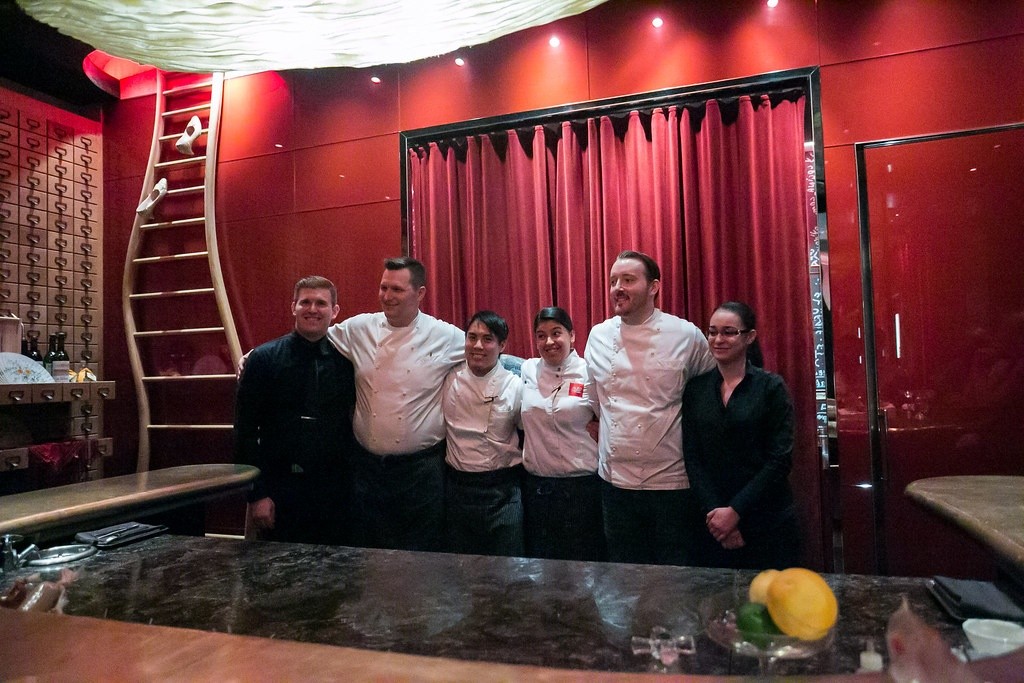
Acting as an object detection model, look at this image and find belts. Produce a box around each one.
[351,436,447,466]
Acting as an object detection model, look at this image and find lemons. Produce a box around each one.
[734,566,838,649]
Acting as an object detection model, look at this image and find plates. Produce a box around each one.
[695,586,840,658]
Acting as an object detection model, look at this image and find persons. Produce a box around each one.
[584,251,716,566]
[232,277,356,545]
[237,256,466,552]
[441,310,599,557]
[520,307,599,560]
[681,301,796,571]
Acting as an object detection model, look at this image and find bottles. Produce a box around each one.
[21,332,69,383]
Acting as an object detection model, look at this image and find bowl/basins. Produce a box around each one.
[963,617,1024,655]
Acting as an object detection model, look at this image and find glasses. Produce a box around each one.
[706,326,751,340]
